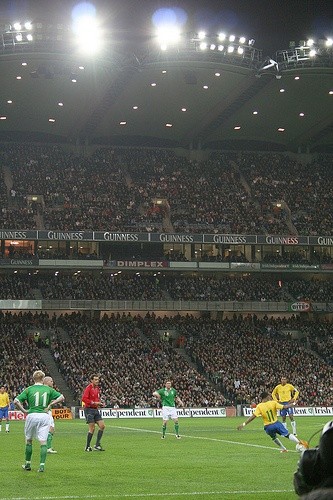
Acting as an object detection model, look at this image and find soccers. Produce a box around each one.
[295,443,305,452]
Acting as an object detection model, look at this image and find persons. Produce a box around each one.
[0,143,333,500]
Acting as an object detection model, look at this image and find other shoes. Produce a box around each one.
[37,465,44,473]
[176,435,181,439]
[5,430,9,433]
[281,449,288,453]
[85,446,94,451]
[94,446,105,451]
[161,436,165,440]
[299,439,307,447]
[293,432,298,437]
[24,463,32,470]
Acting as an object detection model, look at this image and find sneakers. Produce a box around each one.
[46,447,58,453]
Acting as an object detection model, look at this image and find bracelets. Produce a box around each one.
[243,422,246,427]
[293,402,296,405]
